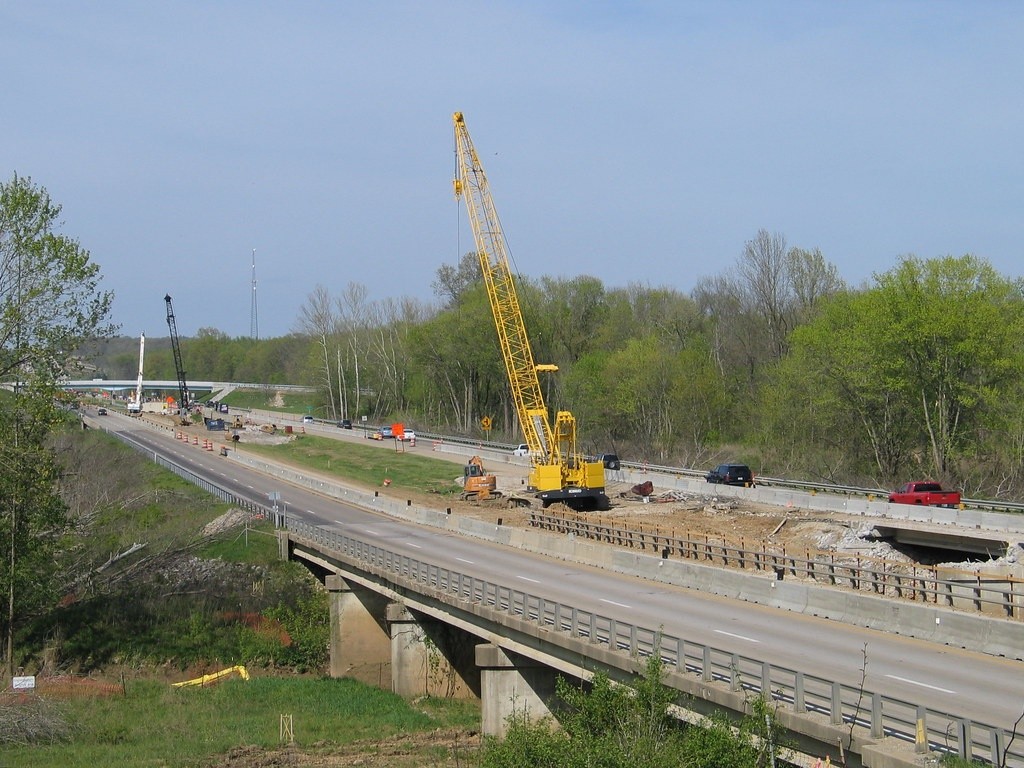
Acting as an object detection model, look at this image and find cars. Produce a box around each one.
[98,409,107,416]
[377,426,395,438]
[303,415,314,424]
[397,428,416,441]
[336,419,352,430]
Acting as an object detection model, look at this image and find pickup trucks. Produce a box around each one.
[888,480,961,509]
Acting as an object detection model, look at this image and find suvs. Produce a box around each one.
[706,464,755,488]
[598,453,620,470]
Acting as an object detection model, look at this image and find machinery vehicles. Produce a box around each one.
[461,455,504,502]
[448,111,611,513]
[126,330,146,414]
[163,293,194,417]
[230,413,244,429]
